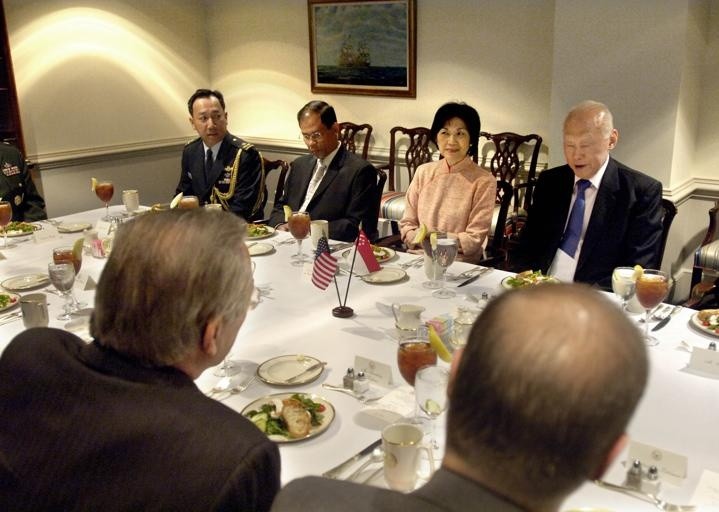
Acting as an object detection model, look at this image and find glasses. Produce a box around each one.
[298,131,321,142]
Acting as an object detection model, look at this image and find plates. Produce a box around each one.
[500,274,562,292]
[361,267,406,284]
[691,307,719,337]
[238,393,335,444]
[146,189,277,257]
[255,354,324,387]
[342,247,396,264]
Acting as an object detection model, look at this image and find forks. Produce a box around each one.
[210,376,254,400]
[593,479,695,511]
[454,266,481,280]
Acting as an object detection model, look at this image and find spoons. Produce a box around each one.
[207,376,230,397]
[638,303,671,323]
[344,447,387,483]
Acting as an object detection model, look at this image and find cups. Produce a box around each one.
[382,424,434,490]
[311,220,329,247]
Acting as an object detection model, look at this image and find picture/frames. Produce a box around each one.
[305,0,415,99]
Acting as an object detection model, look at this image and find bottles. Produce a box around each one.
[354,372,367,392]
[452,293,498,350]
[626,458,642,490]
[640,466,660,495]
[343,368,355,388]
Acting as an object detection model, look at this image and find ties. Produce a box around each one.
[206,149,213,177]
[298,159,327,214]
[559,179,591,258]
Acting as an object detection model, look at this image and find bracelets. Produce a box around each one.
[274,222,284,229]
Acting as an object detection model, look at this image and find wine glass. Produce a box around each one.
[420,223,456,302]
[637,269,670,347]
[288,211,310,258]
[612,266,635,317]
[398,336,438,391]
[415,365,448,448]
[0,177,141,333]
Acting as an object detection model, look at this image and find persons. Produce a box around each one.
[508,99,665,293]
[268,99,377,244]
[0,140,47,224]
[174,89,266,224]
[398,101,498,265]
[268,282,651,511]
[0,206,281,511]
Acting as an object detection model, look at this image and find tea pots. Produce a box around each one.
[393,303,425,332]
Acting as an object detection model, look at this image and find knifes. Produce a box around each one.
[286,361,326,383]
[322,432,385,481]
[652,305,682,331]
[457,267,494,288]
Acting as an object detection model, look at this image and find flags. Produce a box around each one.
[310,237,338,291]
[346,230,381,278]
